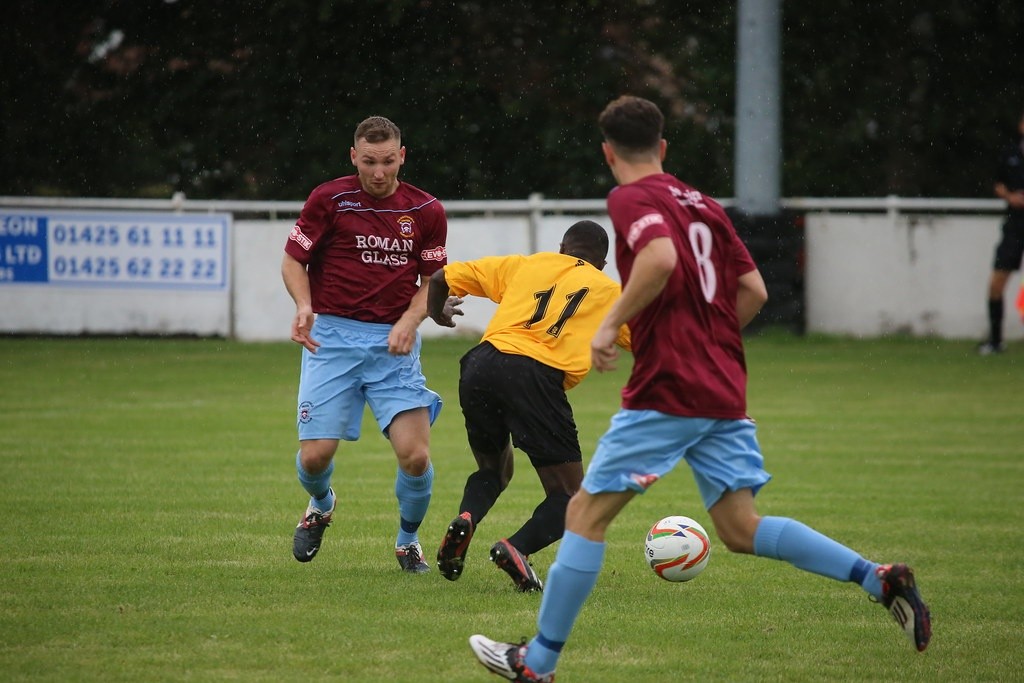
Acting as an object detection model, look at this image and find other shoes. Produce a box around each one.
[980,342,1002,353]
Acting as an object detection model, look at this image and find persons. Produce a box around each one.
[428,220,632,593]
[281,114,446,574]
[468,94,930,682]
[976,104,1024,357]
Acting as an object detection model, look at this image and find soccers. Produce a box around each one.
[644,515,711,582]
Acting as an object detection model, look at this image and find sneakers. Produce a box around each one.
[395,539,431,573]
[437,512,474,582]
[469,634,557,683]
[868,564,932,652]
[489,539,543,592]
[293,487,336,562]
